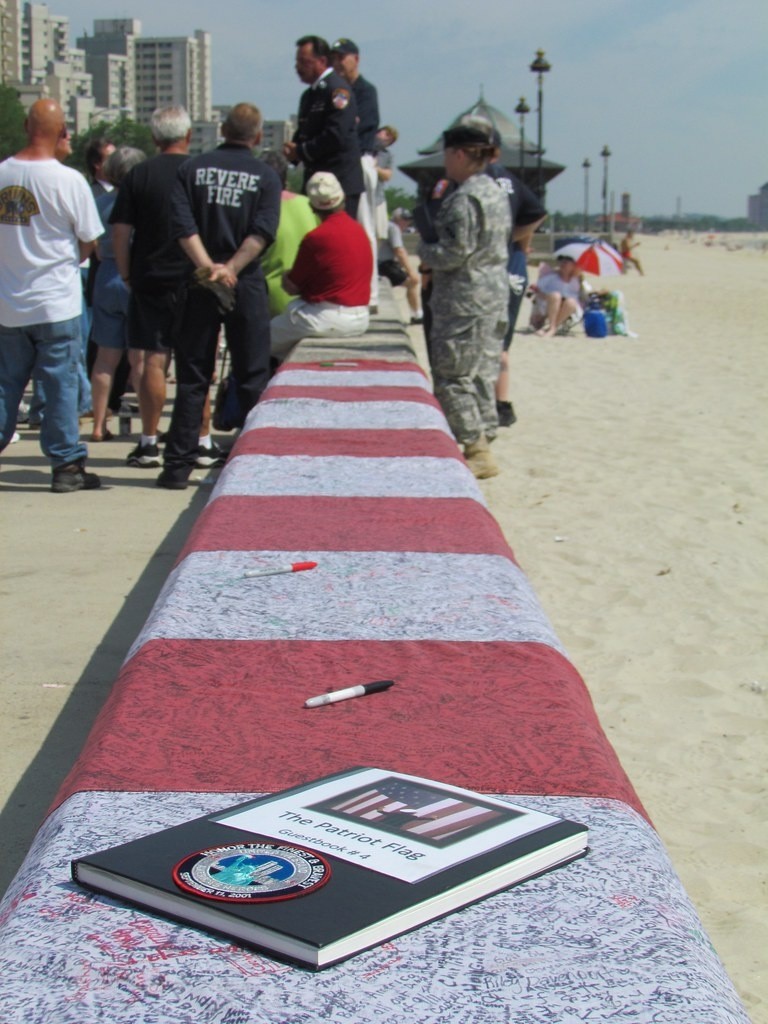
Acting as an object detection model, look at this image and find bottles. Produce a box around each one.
[582,301,607,337]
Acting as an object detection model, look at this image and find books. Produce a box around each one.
[71,766,590,973]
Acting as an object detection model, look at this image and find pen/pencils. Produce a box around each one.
[244,562,317,577]
[320,361,358,367]
[303,680,394,709]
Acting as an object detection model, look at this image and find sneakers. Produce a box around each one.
[156,465,193,489]
[127,441,160,467]
[52,456,100,492]
[194,438,229,468]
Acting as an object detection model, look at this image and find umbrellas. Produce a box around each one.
[554,235,622,276]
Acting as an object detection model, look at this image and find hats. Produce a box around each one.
[556,255,576,262]
[330,39,359,56]
[307,171,345,209]
[444,126,489,146]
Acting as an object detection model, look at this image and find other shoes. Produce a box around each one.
[17,400,32,422]
[30,424,41,430]
[411,315,423,325]
[463,442,499,478]
[10,433,20,443]
[112,401,139,418]
[498,399,516,426]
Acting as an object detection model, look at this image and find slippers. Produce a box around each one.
[91,431,113,442]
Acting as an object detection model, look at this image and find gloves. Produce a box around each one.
[193,266,241,315]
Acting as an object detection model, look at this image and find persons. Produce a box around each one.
[0,98,374,493]
[376,807,437,831]
[210,856,255,887]
[529,256,585,338]
[622,229,643,275]
[383,206,424,325]
[411,114,546,477]
[282,34,395,312]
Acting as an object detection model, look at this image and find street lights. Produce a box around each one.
[582,158,592,233]
[529,49,552,201]
[513,95,530,183]
[601,143,611,232]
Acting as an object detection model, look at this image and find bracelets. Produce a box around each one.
[124,276,130,281]
[417,265,432,274]
